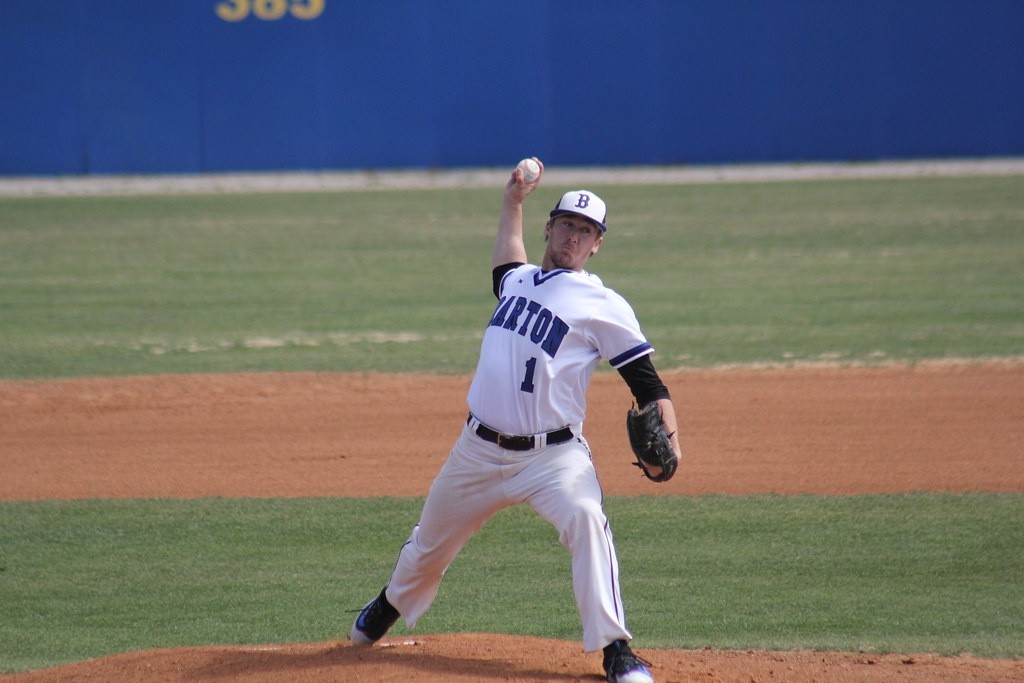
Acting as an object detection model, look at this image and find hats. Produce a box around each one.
[547,190,608,232]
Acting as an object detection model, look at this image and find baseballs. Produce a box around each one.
[516,158,540,184]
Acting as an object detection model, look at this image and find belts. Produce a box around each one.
[467,414,574,452]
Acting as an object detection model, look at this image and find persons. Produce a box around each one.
[349,156,684,683]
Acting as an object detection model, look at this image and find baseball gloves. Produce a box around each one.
[626,399,679,482]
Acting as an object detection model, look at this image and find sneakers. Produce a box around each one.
[603,639,655,683]
[350,583,402,647]
